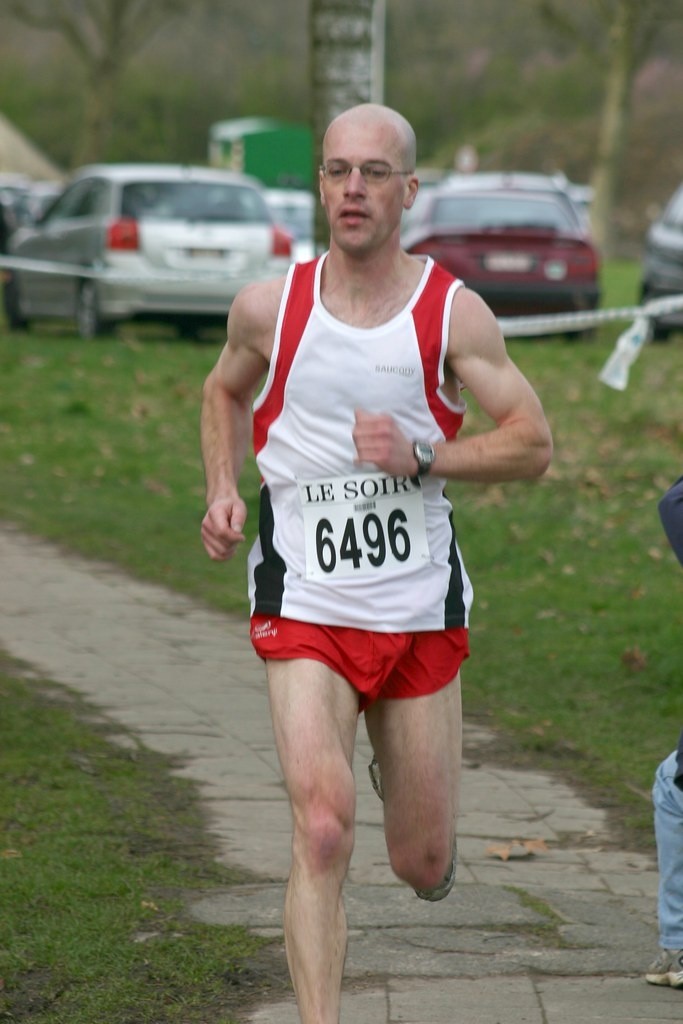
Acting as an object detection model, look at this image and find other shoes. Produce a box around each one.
[368,751,456,902]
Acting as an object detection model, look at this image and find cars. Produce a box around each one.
[396,169,595,236]
[637,183,683,345]
[400,191,604,318]
[0,176,60,245]
[0,161,294,342]
[262,188,314,266]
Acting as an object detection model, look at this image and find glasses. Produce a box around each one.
[320,159,411,186]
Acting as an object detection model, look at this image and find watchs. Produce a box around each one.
[410,439,434,488]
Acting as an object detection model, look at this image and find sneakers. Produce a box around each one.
[645,949,683,988]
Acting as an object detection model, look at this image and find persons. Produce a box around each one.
[198,105,553,1024]
[643,476,682,989]
